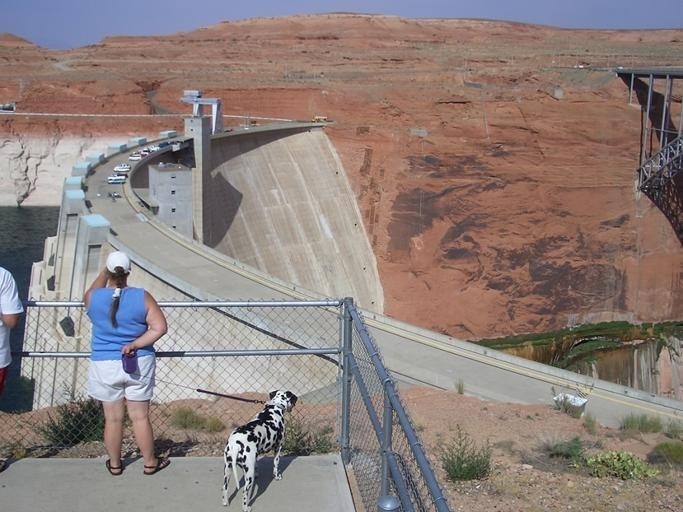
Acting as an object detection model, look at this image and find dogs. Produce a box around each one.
[222,390,297,512]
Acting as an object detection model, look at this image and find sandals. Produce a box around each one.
[142,456,170,476]
[104,457,123,476]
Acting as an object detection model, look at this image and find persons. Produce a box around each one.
[1,266,25,471]
[82,250,170,477]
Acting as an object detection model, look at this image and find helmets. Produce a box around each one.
[104,250,131,276]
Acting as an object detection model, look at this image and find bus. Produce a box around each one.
[128,154,142,161]
[108,176,126,185]
[113,163,131,172]
[138,149,149,156]
